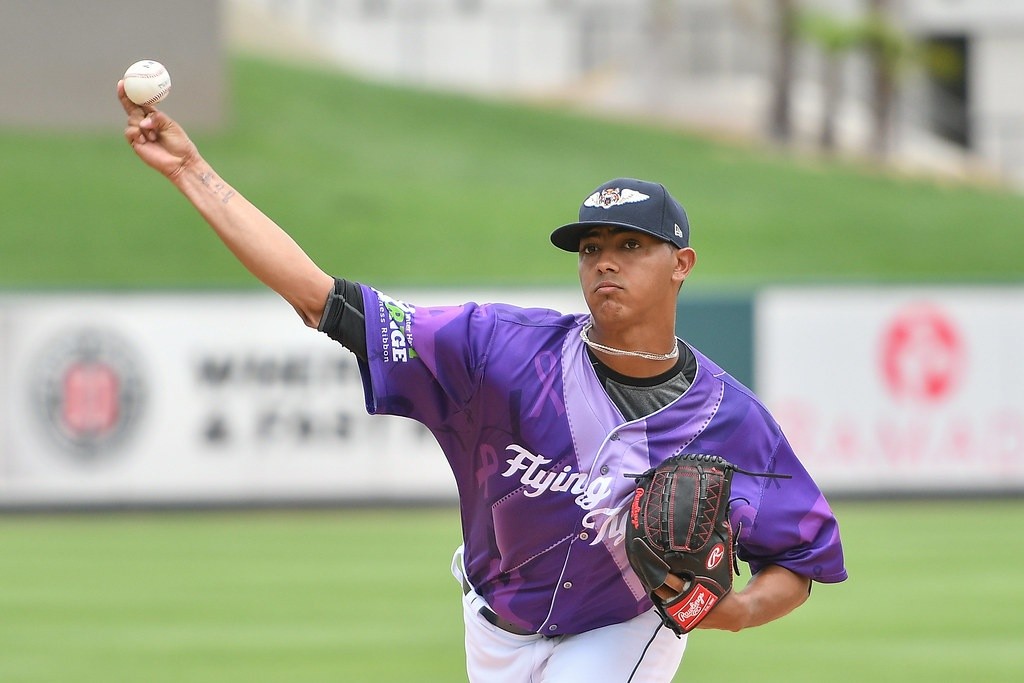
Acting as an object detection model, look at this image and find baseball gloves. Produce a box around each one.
[623,453,742,638]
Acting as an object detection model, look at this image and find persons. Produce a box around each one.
[116,80,848,683]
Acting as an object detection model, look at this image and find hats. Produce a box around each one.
[551,177,690,254]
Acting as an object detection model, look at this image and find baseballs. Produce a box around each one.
[123,59,173,109]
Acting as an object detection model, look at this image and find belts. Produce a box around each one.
[459,553,538,636]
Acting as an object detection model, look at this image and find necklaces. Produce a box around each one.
[580,320,679,360]
